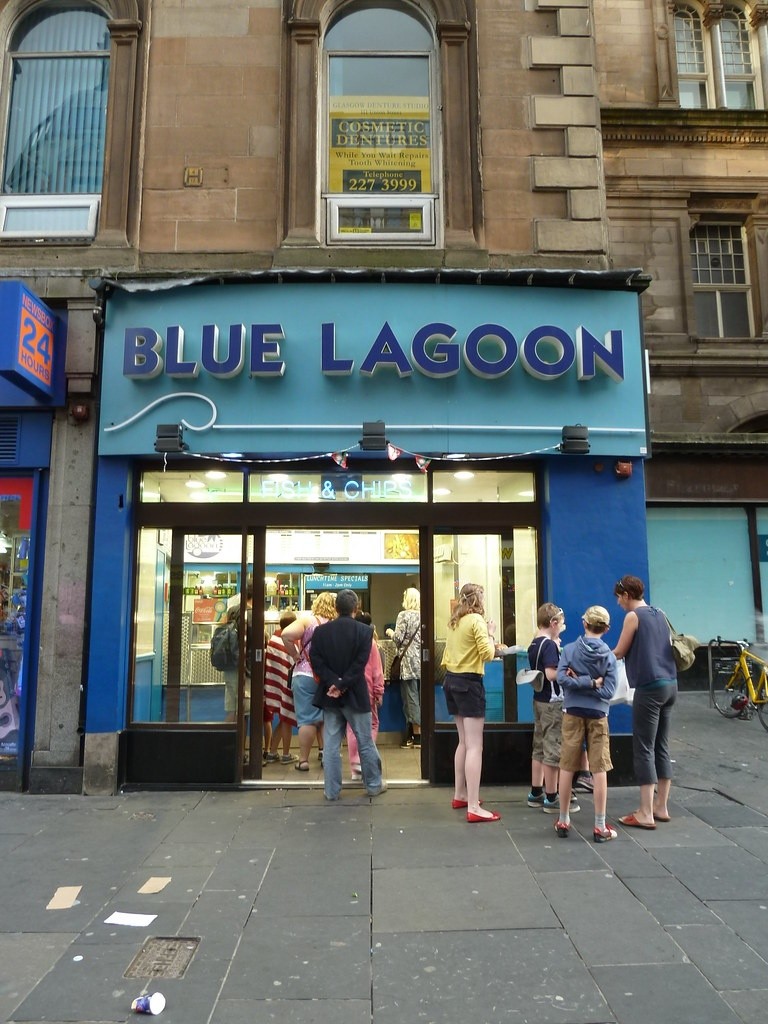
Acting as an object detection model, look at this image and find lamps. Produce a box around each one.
[361,420,386,450]
[154,422,186,454]
[559,423,592,453]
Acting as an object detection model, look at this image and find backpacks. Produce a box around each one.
[208,605,252,671]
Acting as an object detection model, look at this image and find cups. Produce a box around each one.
[132,992,166,1015]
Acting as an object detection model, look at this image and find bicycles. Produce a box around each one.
[709,637,768,731]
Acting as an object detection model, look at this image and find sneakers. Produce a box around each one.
[542,794,581,813]
[594,823,617,843]
[528,791,561,808]
[554,819,571,838]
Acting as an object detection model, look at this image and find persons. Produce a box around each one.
[527,604,580,814]
[265,611,299,764]
[263,632,273,758]
[346,610,384,780]
[612,576,678,829]
[309,589,388,800]
[441,583,508,822]
[552,635,595,802]
[223,578,253,762]
[281,592,338,771]
[555,606,618,843]
[385,588,421,749]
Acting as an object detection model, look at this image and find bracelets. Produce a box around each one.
[489,635,494,640]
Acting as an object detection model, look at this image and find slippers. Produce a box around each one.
[617,816,656,829]
[634,809,672,822]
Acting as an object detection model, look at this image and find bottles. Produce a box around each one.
[280,598,299,611]
[213,584,235,595]
[279,584,299,595]
[184,585,203,594]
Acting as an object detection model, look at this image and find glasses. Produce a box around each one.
[617,578,626,592]
[550,608,564,623]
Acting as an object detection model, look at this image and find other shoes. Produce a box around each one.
[572,774,594,793]
[571,791,578,801]
[452,798,484,810]
[401,738,421,749]
[323,788,339,801]
[467,811,501,823]
[350,771,363,781]
[368,780,388,796]
[244,750,324,771]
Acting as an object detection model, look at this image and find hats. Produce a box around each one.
[516,668,544,693]
[582,605,610,630]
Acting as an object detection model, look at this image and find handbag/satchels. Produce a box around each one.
[391,655,401,680]
[610,657,630,706]
[287,665,293,689]
[655,608,701,671]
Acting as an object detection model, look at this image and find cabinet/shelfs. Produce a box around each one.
[183,571,304,625]
